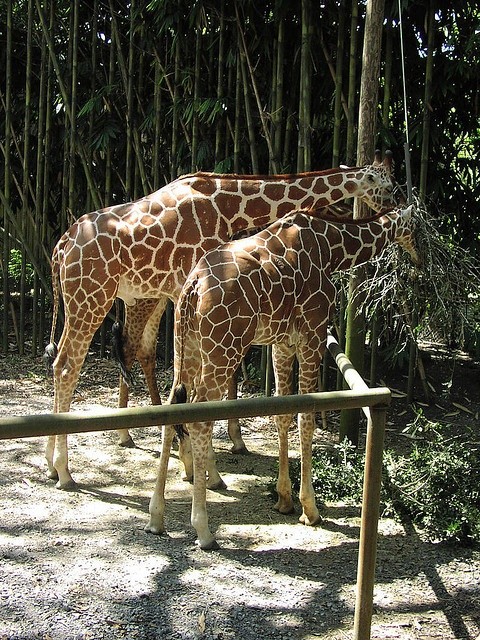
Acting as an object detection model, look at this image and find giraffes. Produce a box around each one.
[112,294,256,455]
[42,149,396,490]
[144,204,420,546]
